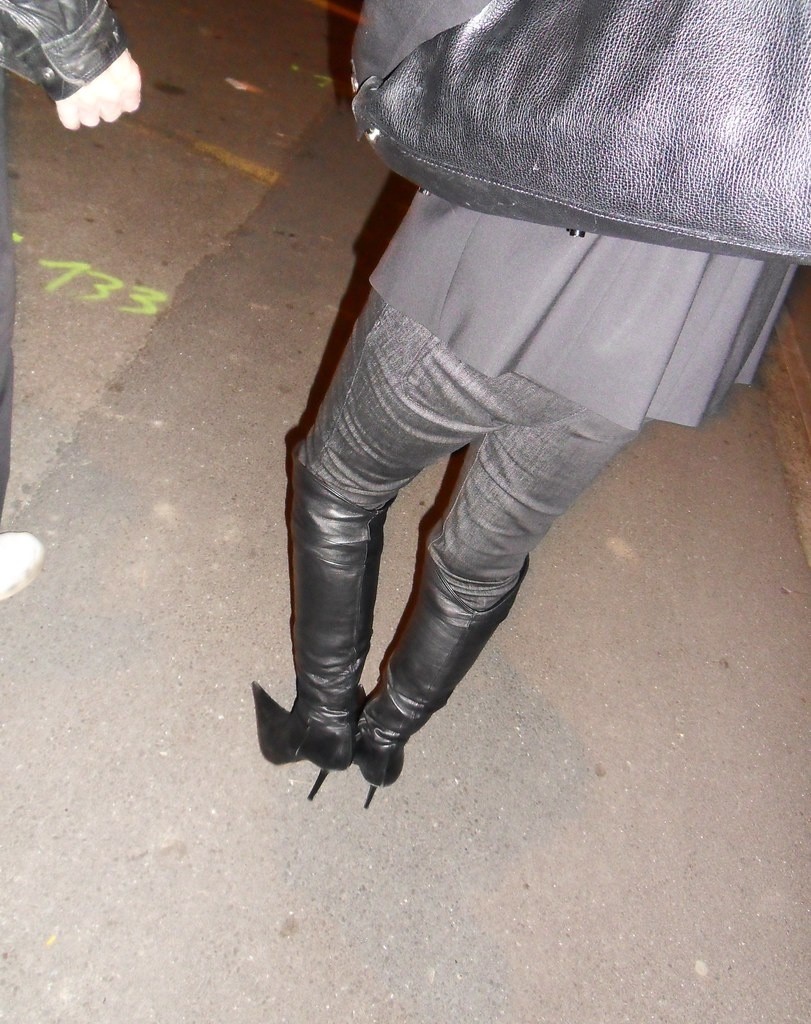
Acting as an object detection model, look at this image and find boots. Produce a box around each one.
[252,438,400,802]
[353,552,529,807]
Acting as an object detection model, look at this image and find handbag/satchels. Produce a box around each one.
[352,0,811,256]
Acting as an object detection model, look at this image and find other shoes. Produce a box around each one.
[0,530,44,600]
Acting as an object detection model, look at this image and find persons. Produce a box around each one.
[252,2,809,809]
[1,0,145,601]
